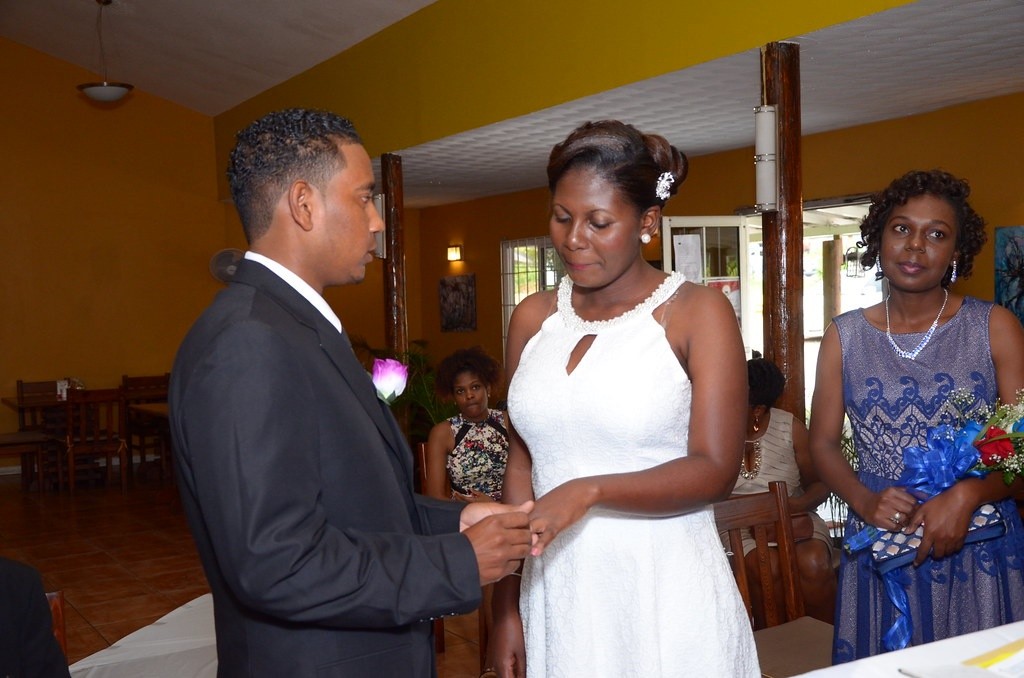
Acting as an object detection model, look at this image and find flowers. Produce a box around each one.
[839,383,1024,558]
[370,358,409,406]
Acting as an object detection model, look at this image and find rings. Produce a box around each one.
[893,511,902,523]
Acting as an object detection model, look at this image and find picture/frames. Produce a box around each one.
[438,272,477,333]
[990,223,1024,328]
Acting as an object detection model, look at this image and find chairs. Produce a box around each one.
[712,480,843,678]
[0,371,180,496]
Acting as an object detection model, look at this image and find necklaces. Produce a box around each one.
[885,289,948,361]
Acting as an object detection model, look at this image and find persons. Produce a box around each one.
[482,118,751,678]
[2,558,70,678]
[426,340,508,504]
[716,356,837,625]
[810,164,1024,668]
[167,113,534,678]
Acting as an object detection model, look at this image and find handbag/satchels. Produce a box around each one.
[857,496,1008,574]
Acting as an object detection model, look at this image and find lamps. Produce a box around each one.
[447,244,462,262]
